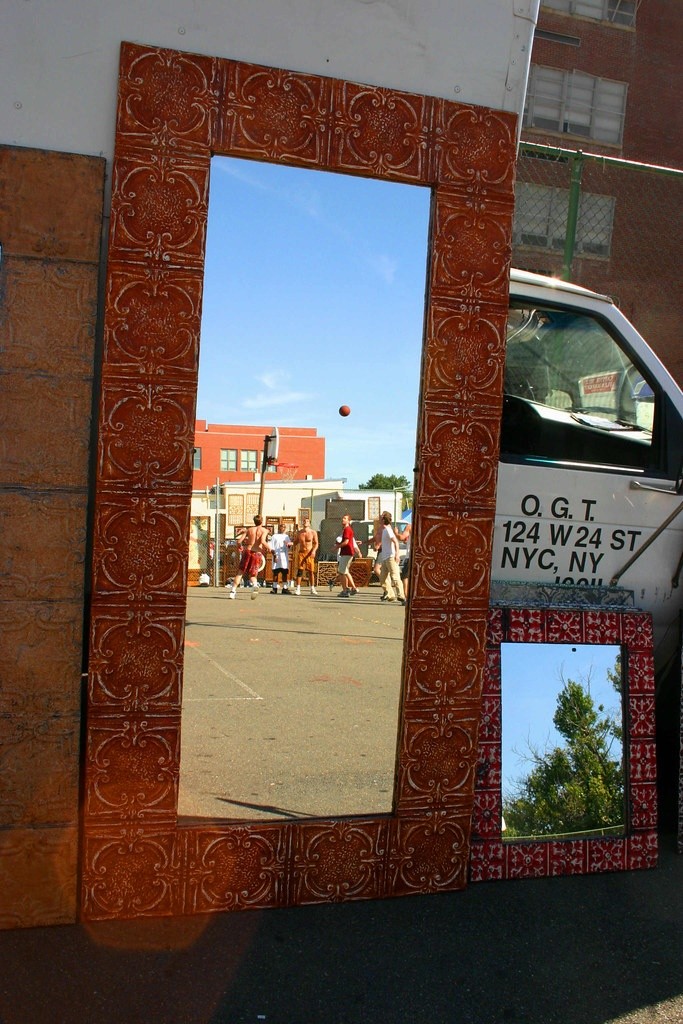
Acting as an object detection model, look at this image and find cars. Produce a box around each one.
[209,537,245,566]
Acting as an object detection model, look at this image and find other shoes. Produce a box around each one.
[337,590,350,597]
[329,579,335,591]
[397,596,405,602]
[350,587,359,595]
[402,601,406,606]
[312,590,317,594]
[388,597,396,601]
[294,591,301,595]
[380,592,388,600]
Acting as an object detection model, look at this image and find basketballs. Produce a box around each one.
[339,405,350,417]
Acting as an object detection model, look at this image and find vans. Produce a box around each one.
[337,520,412,576]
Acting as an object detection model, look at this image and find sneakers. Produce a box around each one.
[251,586,259,600]
[270,588,277,594]
[282,588,292,594]
[229,591,236,598]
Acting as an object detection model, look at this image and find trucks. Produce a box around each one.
[0,2,683,697]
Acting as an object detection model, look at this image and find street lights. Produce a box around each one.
[210,476,227,588]
[394,483,410,528]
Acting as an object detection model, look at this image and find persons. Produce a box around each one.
[330,516,362,597]
[356,511,412,604]
[229,514,318,599]
